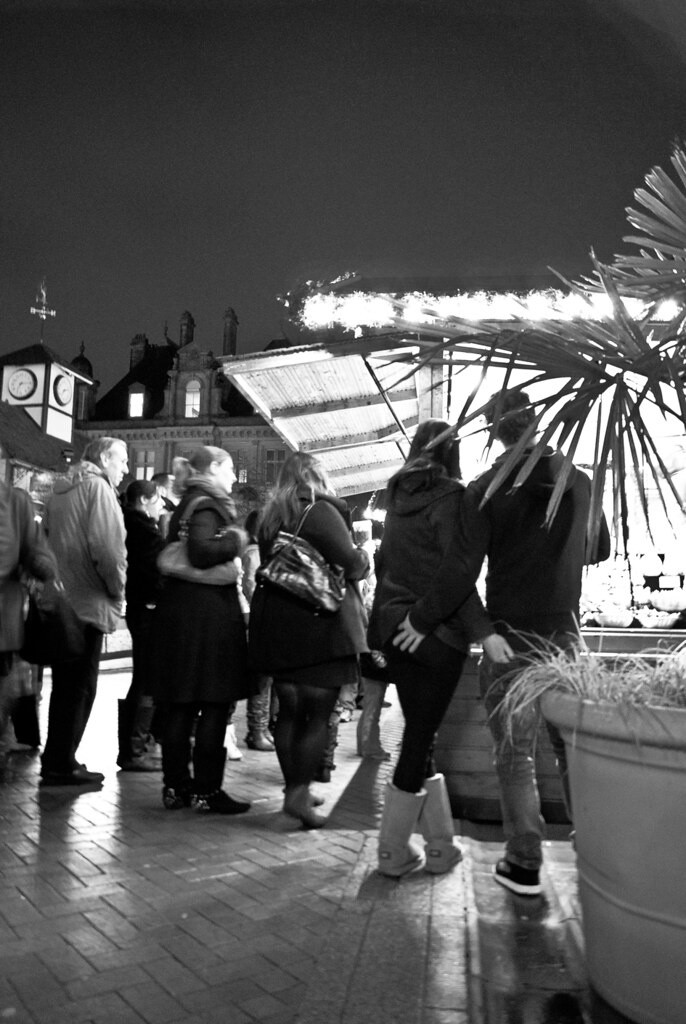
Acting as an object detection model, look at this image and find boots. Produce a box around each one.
[243,675,276,752]
[116,697,164,772]
[190,745,252,815]
[417,772,463,874]
[310,705,344,782]
[376,779,428,879]
[356,676,392,759]
[223,723,243,760]
[158,737,196,809]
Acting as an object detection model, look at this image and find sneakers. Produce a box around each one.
[491,858,542,895]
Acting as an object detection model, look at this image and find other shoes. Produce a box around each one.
[280,774,330,828]
[381,698,391,707]
[340,706,355,723]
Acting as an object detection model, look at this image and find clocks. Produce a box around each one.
[7,368,39,401]
[55,374,73,407]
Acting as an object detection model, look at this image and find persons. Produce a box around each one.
[0,387,618,898]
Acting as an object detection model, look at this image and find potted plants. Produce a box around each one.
[317,143,685,1024]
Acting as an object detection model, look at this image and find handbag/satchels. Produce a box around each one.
[19,594,68,668]
[156,532,240,585]
[254,531,349,614]
[8,679,41,746]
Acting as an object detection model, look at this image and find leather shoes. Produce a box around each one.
[39,762,107,786]
